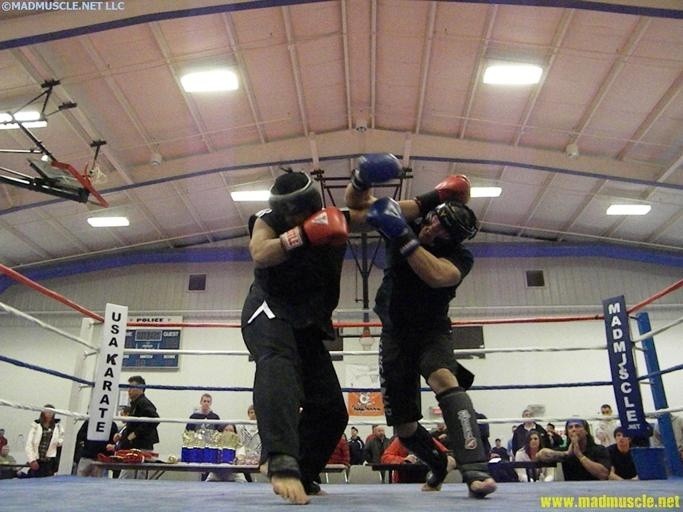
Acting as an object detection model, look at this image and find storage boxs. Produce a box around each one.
[181,446,236,465]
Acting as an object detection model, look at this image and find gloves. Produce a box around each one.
[367,195,422,257]
[414,174,472,217]
[278,203,349,250]
[349,153,403,191]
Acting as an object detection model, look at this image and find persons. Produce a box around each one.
[342,151,497,499]
[104,404,131,478]
[653,413,683,465]
[0,445,17,479]
[238,403,263,464]
[203,420,238,481]
[73,417,118,477]
[117,374,160,479]
[237,165,472,506]
[0,428,7,451]
[324,395,643,482]
[24,404,65,477]
[185,394,221,432]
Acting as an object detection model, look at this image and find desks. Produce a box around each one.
[0,464,31,479]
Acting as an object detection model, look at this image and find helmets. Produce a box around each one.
[269,171,322,215]
[423,200,479,245]
